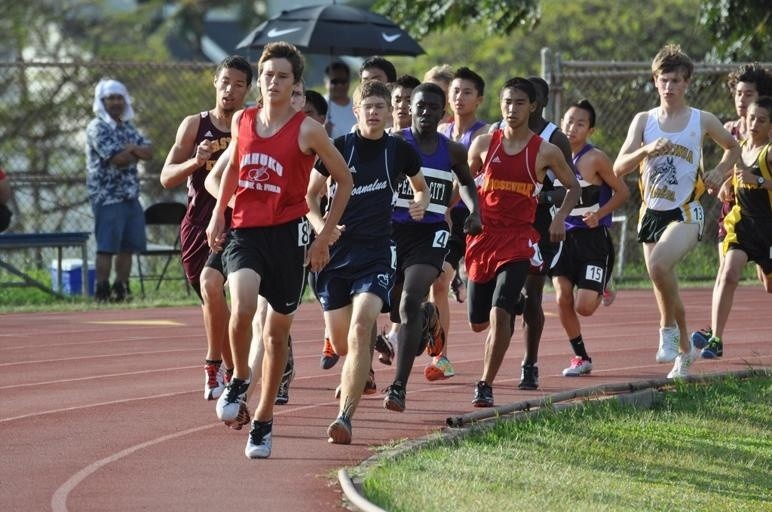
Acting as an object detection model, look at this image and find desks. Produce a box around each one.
[0,231,91,304]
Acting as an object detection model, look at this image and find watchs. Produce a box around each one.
[757,175,764,188]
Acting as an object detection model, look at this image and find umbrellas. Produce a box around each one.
[236,0,428,119]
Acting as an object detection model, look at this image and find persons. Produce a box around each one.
[161,56,253,400]
[546,98,626,377]
[85,80,154,301]
[692,64,772,349]
[205,77,334,430]
[320,56,491,399]
[0,169,11,205]
[700,96,772,359]
[322,61,357,144]
[488,77,576,391]
[613,46,741,379]
[305,80,430,443]
[464,77,583,407]
[380,82,482,412]
[205,44,353,457]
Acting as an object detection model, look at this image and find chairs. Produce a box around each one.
[136,202,192,299]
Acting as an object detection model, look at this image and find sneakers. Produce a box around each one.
[321,335,406,411]
[602,276,617,306]
[563,356,592,376]
[328,417,352,444]
[95,282,132,303]
[204,361,296,458]
[656,320,723,378]
[516,293,525,315]
[450,264,466,302]
[423,301,455,381]
[471,381,494,407]
[519,366,539,390]
